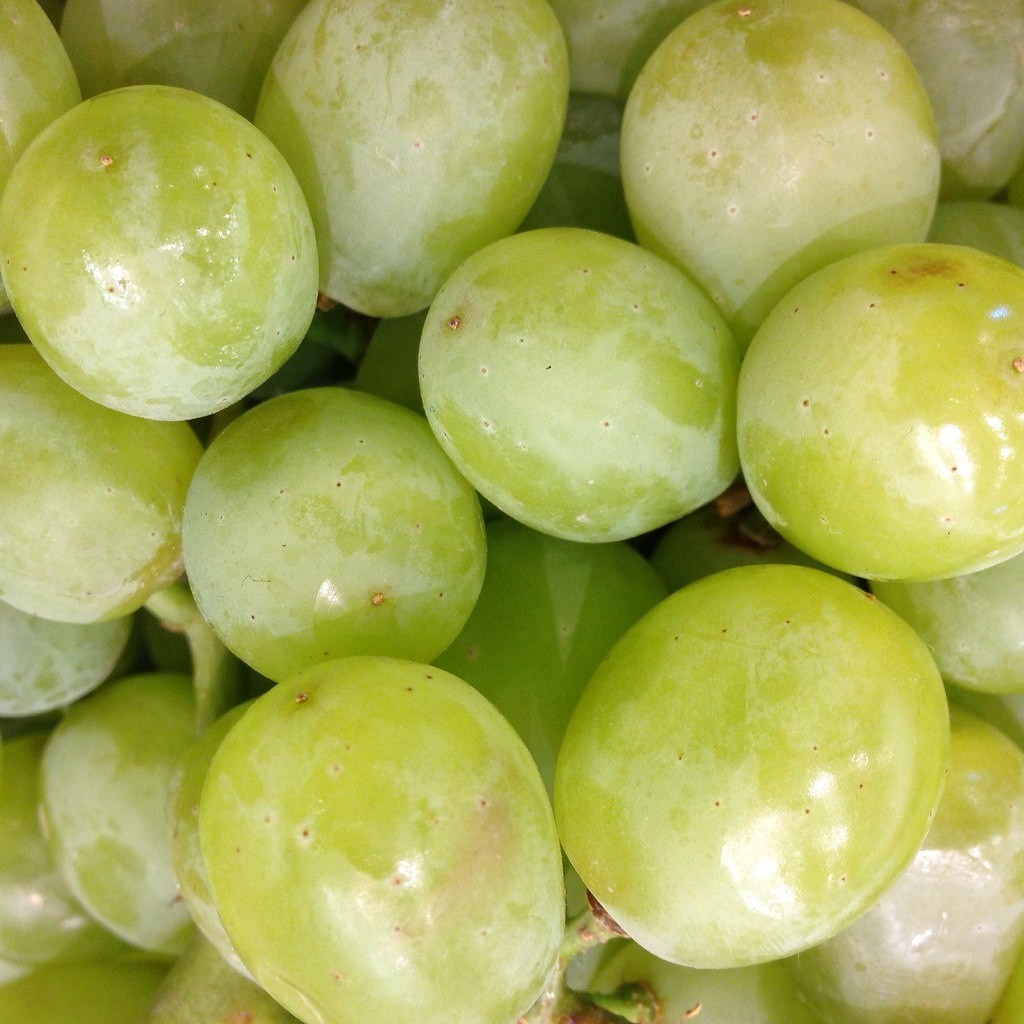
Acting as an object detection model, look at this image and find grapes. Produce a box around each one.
[0,0,1024,1024]
